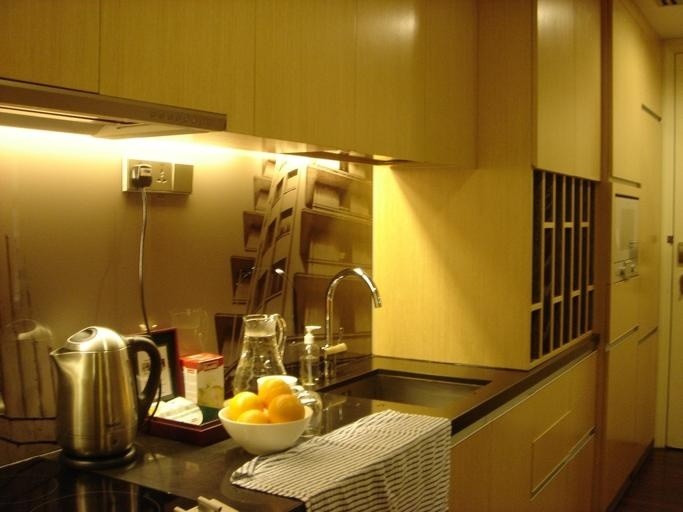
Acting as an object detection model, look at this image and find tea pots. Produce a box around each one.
[232,313,288,395]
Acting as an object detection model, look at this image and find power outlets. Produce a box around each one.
[121,157,172,194]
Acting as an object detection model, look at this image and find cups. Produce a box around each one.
[255,375,299,394]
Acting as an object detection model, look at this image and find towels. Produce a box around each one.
[229,408,452,512]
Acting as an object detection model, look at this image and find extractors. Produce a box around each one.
[0,79,226,147]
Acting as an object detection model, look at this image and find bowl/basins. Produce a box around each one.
[216,404,314,456]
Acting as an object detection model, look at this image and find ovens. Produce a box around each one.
[610,187,639,281]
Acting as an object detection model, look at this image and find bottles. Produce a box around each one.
[295,384,323,438]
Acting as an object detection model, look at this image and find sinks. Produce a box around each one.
[319,366,495,413]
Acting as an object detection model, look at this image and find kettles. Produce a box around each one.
[1,320,57,419]
[46,326,160,473]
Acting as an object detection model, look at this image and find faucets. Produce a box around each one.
[319,267,383,381]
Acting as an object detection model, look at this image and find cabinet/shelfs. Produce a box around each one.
[356,0,480,170]
[370,0,603,372]
[488,343,598,512]
[0,0,101,136]
[599,0,660,511]
[254,0,358,152]
[99,0,255,136]
